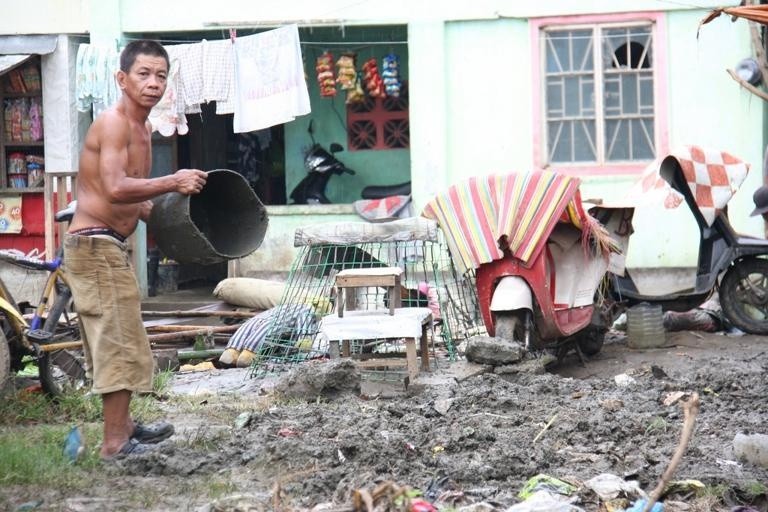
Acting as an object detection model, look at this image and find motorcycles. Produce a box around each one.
[289,119,411,206]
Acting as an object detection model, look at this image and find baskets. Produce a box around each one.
[243,226,490,382]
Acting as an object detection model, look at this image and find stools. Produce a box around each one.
[331,267,404,318]
[321,307,434,382]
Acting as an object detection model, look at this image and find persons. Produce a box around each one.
[61,39,209,462]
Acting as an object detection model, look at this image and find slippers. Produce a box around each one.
[128,419,175,445]
[98,439,168,464]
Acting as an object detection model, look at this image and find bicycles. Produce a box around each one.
[0,200,109,408]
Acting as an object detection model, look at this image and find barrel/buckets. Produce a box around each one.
[626,301,666,350]
[146,169,269,270]
[152,261,179,293]
